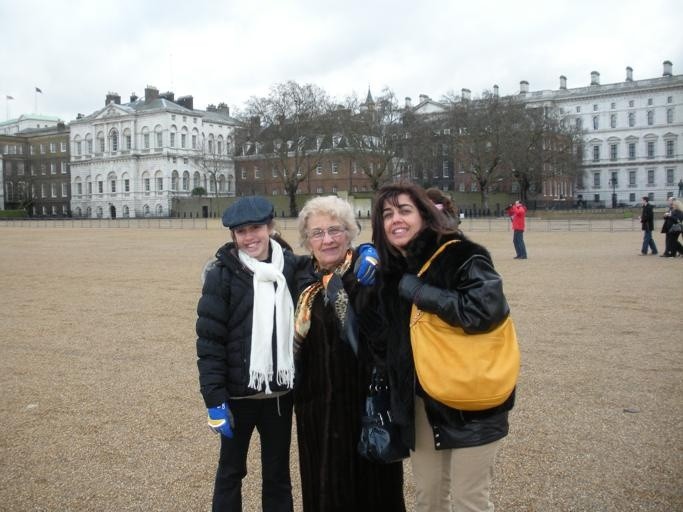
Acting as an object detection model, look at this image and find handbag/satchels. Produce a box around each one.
[357,365,411,464]
[668,223,683,233]
[410,304,521,412]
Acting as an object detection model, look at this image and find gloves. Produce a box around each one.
[399,273,423,301]
[353,243,381,287]
[207,401,235,439]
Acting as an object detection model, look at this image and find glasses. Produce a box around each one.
[308,227,343,238]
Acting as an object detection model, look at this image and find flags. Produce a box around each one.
[7,95,14,101]
[36,87,42,93]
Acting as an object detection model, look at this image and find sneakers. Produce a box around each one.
[640,251,677,257]
[514,255,527,259]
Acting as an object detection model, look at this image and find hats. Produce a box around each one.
[222,196,275,229]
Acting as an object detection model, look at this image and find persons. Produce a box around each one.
[660,196,676,257]
[506,197,529,260]
[194,196,380,510]
[638,196,659,255]
[664,200,683,258]
[291,196,411,510]
[425,186,462,229]
[369,178,517,511]
